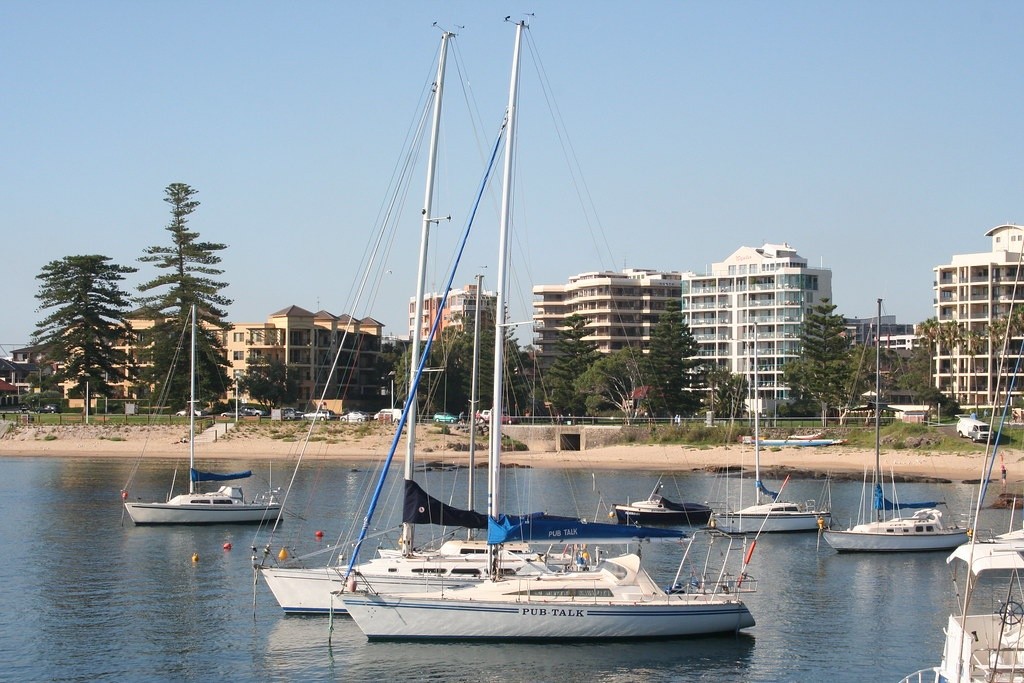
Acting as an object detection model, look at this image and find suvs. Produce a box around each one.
[240,405,267,417]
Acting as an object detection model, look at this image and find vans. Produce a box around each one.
[374,408,404,422]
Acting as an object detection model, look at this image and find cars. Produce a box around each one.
[175,406,202,417]
[301,409,335,422]
[481,409,518,426]
[221,407,246,419]
[432,412,459,424]
[339,410,371,423]
[36,404,63,415]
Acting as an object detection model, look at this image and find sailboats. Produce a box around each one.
[815,298,972,553]
[900,232,1024,683]
[706,320,834,536]
[250,16,604,612]
[330,13,757,642]
[120,301,283,526]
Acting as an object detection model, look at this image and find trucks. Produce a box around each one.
[956,418,995,444]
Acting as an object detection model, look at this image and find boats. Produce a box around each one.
[612,472,714,526]
[788,429,828,440]
[751,438,848,447]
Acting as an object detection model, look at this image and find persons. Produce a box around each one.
[457,409,480,426]
[970,408,1019,423]
[638,408,649,422]
[675,412,700,426]
[556,413,572,425]
[998,452,1009,489]
